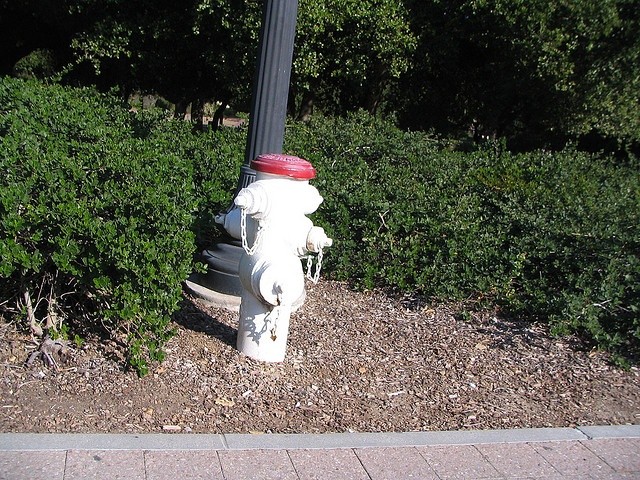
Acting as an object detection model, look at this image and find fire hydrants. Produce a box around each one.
[213,151,333,364]
